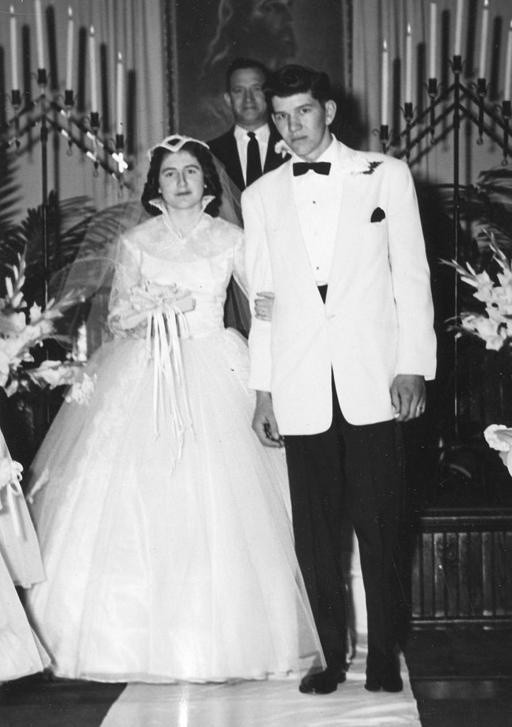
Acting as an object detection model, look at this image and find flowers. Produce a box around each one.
[340,155,384,178]
[438,224,512,352]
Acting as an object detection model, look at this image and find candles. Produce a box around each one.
[2,0,125,137]
[380,0,511,124]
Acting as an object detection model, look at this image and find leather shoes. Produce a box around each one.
[364,657,403,692]
[299,666,346,696]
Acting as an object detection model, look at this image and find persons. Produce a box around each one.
[206,57,291,339]
[243,63,443,696]
[23,135,280,686]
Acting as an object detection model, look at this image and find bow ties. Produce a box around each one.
[293,162,332,176]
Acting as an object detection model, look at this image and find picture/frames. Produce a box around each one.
[159,2,353,142]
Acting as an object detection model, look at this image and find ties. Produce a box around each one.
[245,132,262,187]
